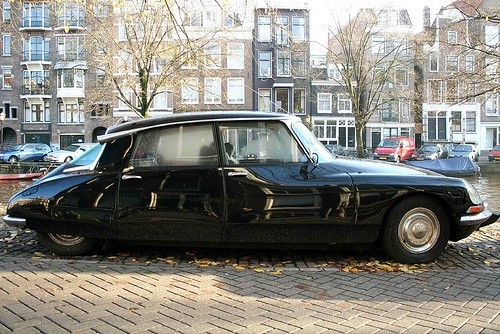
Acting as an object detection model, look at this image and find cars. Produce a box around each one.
[487,144,500,162]
[4,111,500,263]
[0,142,52,164]
[325,144,343,155]
[410,143,447,161]
[448,143,479,162]
[43,143,98,166]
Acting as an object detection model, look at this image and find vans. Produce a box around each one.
[373,135,415,164]
